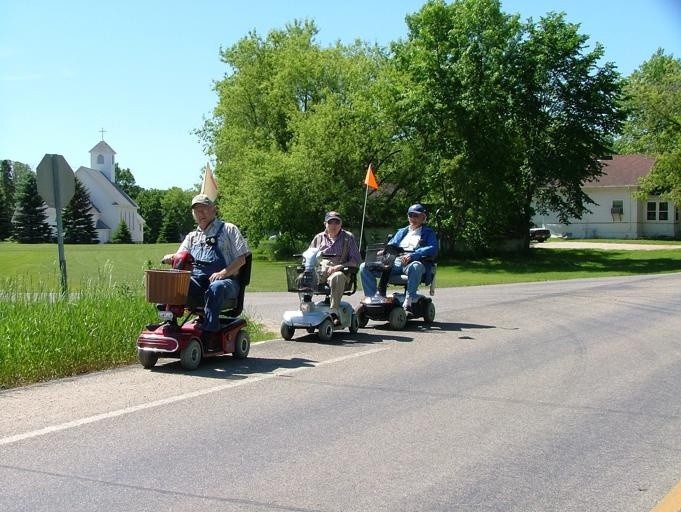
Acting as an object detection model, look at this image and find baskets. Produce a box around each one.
[286,264,318,293]
[365,244,394,272]
[144,269,191,305]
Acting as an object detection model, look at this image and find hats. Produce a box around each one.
[190,194,214,208]
[407,204,426,216]
[324,211,342,225]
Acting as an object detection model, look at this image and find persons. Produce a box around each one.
[298,211,364,326]
[147,194,253,351]
[360,203,440,317]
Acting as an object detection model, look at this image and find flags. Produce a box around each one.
[365,165,379,190]
[201,162,219,202]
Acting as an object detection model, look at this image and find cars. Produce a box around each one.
[529,220,551,242]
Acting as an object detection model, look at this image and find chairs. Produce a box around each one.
[299,262,359,296]
[188,252,253,318]
[384,234,441,288]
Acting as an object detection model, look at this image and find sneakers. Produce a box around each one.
[329,308,340,320]
[403,304,415,317]
